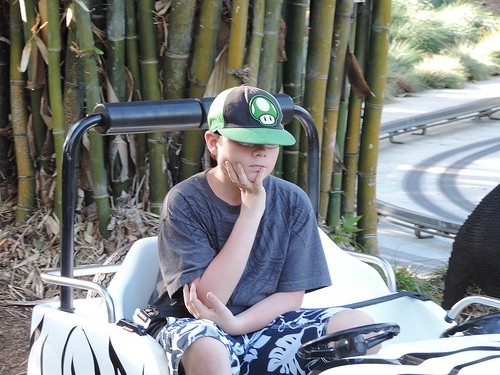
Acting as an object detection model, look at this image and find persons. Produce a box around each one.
[143,87,381,375]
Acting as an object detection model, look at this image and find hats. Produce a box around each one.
[207,84,301,146]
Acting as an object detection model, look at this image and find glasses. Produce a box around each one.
[232,140,280,149]
